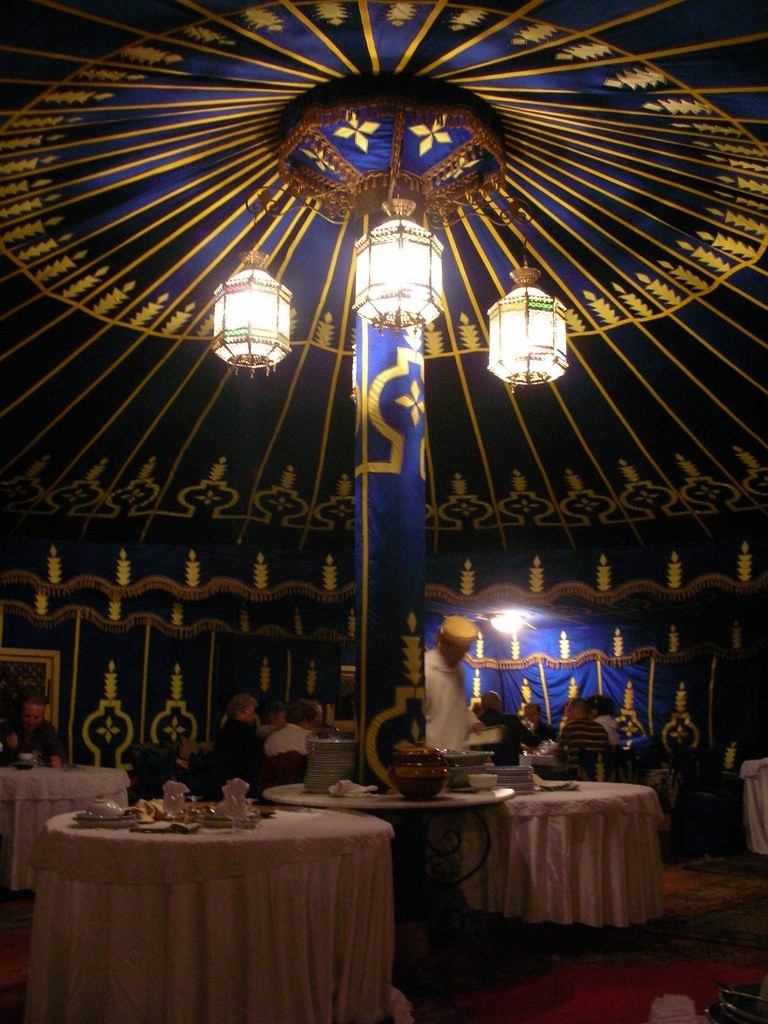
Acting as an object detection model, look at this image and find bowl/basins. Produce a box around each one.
[87,800,119,817]
[467,773,498,791]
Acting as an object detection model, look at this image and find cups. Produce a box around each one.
[232,811,263,833]
[19,753,34,760]
[163,791,196,817]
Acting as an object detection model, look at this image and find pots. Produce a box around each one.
[388,740,451,802]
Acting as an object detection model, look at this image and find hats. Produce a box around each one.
[440,615,478,646]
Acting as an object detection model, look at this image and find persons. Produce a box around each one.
[469,690,622,780]
[380,984,418,1024]
[203,695,278,805]
[0,701,64,768]
[424,615,483,753]
[220,693,340,787]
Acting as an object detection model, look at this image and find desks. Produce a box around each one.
[27,806,397,1024]
[426,779,665,929]
[0,766,131,897]
[262,786,503,991]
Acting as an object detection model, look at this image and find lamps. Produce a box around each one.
[211,215,292,375]
[486,221,569,388]
[352,183,445,331]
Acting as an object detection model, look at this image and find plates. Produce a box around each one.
[303,738,356,794]
[196,815,250,826]
[14,763,33,771]
[492,765,535,795]
[73,813,136,828]
